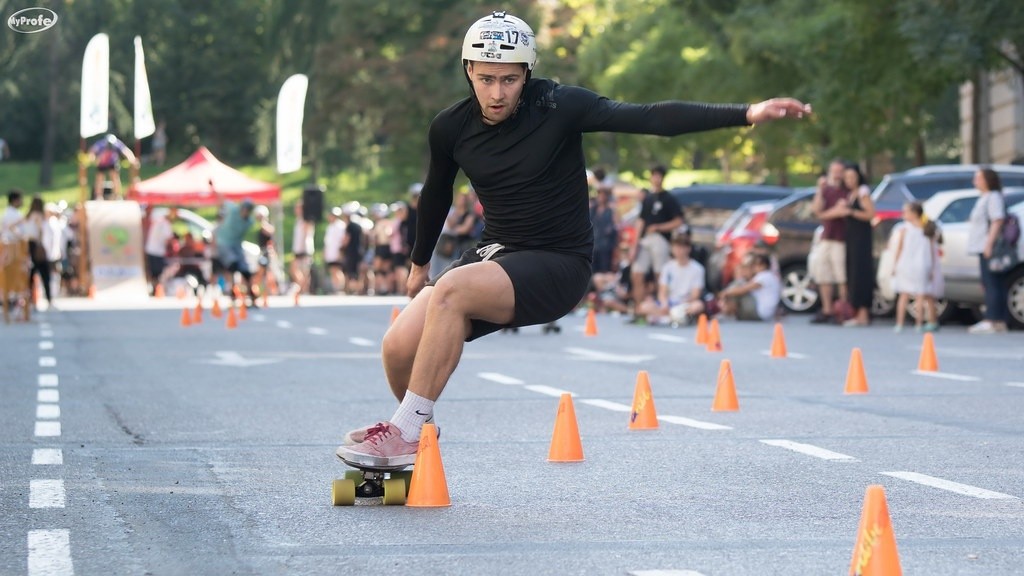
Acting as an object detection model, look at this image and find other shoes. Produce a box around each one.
[969,320,1007,334]
[843,317,870,328]
[923,321,941,331]
[812,314,836,325]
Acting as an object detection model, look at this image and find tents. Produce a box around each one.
[124,147,288,295]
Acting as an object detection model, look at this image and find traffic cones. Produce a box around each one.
[192,303,203,323]
[404,422,453,508]
[238,301,248,320]
[918,332,939,373]
[182,307,192,326]
[628,370,661,430]
[840,347,870,395]
[712,360,740,412]
[703,319,723,350]
[390,306,400,325]
[547,392,587,463]
[87,282,96,300]
[848,485,906,576]
[211,301,221,317]
[695,313,708,345]
[225,304,237,328]
[582,308,599,337]
[154,282,164,299]
[770,324,789,357]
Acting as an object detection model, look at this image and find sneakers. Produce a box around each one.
[336,420,442,466]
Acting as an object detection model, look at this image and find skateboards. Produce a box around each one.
[332,459,415,506]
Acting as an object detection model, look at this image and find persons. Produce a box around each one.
[323,184,423,297]
[292,199,316,295]
[587,163,708,326]
[964,169,1013,334]
[334,10,814,469]
[85,133,143,203]
[889,202,946,332]
[0,188,80,322]
[427,180,487,284]
[712,250,780,320]
[142,179,277,298]
[807,156,873,330]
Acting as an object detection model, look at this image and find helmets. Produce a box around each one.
[460,11,536,70]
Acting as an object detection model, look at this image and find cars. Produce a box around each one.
[616,161,1023,324]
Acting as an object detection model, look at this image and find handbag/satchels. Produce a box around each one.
[999,215,1019,246]
[987,233,1019,271]
